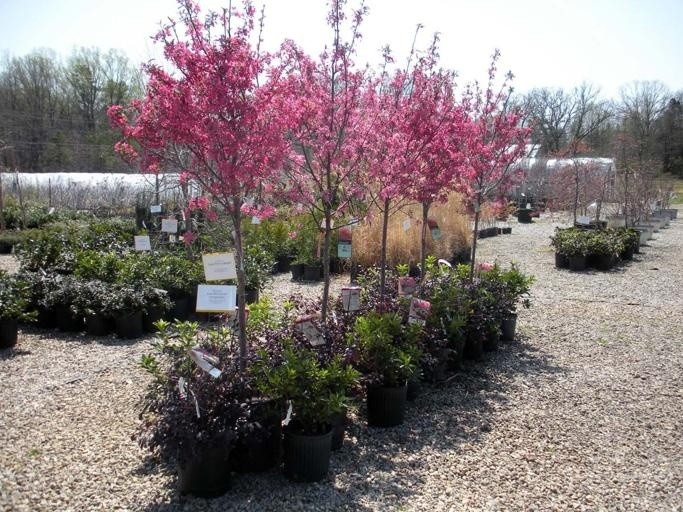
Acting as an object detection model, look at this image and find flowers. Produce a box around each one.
[0,268,176,324]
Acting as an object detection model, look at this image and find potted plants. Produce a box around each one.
[549,224,641,272]
[130,254,538,464]
[290,229,322,280]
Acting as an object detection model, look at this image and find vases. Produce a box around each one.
[0,322,17,351]
[86,320,105,336]
[118,311,144,339]
[177,450,235,499]
[288,418,335,482]
